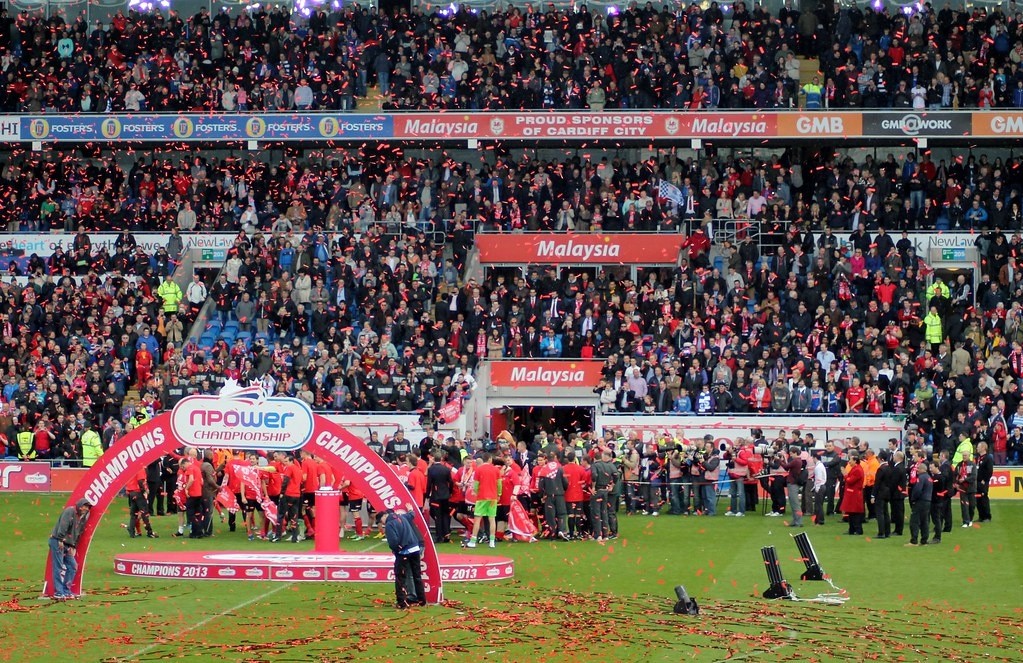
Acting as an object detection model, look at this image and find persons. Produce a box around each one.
[375,502,428,608]
[0,0,1023,110]
[3,136,1023,547]
[47,497,93,598]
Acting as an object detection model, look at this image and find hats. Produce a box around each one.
[375,511,387,524]
[84,502,92,506]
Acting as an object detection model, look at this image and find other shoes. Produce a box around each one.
[172,532,183,536]
[53,592,66,599]
[479,535,487,544]
[556,531,618,542]
[245,526,315,543]
[183,525,189,527]
[148,534,158,537]
[339,527,451,549]
[64,593,74,598]
[640,509,991,548]
[139,533,142,536]
[489,541,495,547]
[210,534,218,538]
[466,542,474,548]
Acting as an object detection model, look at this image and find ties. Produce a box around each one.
[522,455,523,461]
